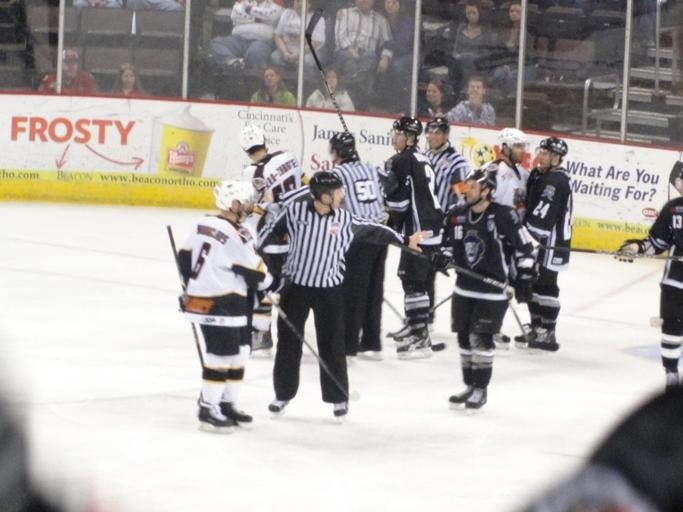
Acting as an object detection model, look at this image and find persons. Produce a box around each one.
[473,128,536,345]
[331,1,394,95]
[269,1,326,82]
[175,177,274,430]
[380,1,418,91]
[247,68,297,107]
[208,0,284,79]
[253,171,433,419]
[317,132,387,360]
[419,118,473,225]
[227,125,305,357]
[613,160,681,395]
[431,168,540,412]
[434,76,496,126]
[36,49,101,92]
[108,63,150,96]
[420,80,450,117]
[442,1,492,87]
[380,115,445,358]
[514,136,572,353]
[488,4,535,88]
[305,69,355,113]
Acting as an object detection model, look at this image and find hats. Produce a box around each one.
[63,49,79,60]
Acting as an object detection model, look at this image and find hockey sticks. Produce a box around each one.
[383,296,445,352]
[508,302,528,341]
[305,8,361,161]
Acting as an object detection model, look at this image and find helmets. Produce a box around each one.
[389,117,422,136]
[330,133,355,160]
[499,128,527,152]
[540,138,568,155]
[463,169,497,190]
[311,172,343,200]
[425,118,450,131]
[240,126,264,151]
[215,180,255,224]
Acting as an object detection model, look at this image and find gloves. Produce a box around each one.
[429,246,452,269]
[514,273,533,303]
[614,239,646,263]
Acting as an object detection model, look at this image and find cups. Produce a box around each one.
[146,117,215,179]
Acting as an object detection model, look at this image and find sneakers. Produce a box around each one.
[394,324,414,341]
[515,329,538,342]
[493,332,510,344]
[198,402,233,427]
[221,401,252,423]
[466,388,487,408]
[269,400,289,412]
[252,329,273,352]
[450,387,473,402]
[361,338,381,352]
[334,403,348,416]
[528,334,558,350]
[397,338,430,351]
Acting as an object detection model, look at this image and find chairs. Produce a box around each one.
[0,5,21,35]
[539,1,585,39]
[131,7,183,44]
[84,44,130,77]
[33,5,75,42]
[538,38,592,73]
[585,2,632,39]
[80,1,127,40]
[1,40,28,73]
[133,47,182,80]
